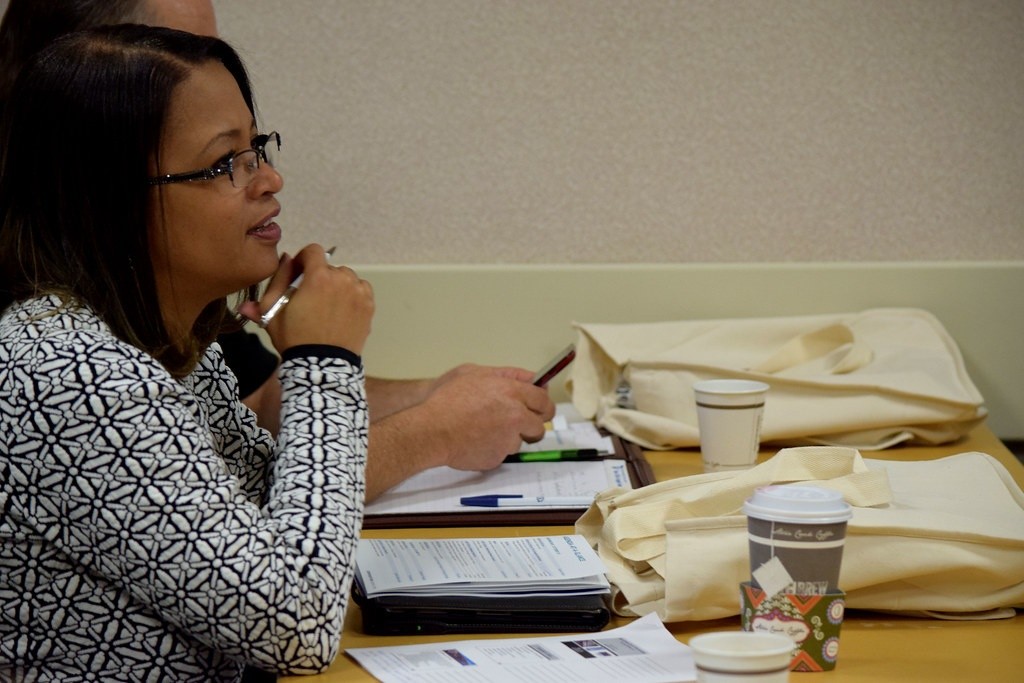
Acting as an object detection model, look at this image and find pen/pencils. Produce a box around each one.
[464,490,597,507]
[257,244,338,330]
[503,446,613,462]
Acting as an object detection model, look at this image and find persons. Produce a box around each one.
[0,0,555,509]
[0,25,375,683]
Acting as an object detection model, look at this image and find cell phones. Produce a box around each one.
[533,343,576,387]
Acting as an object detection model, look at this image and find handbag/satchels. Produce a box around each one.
[574,448,1024,619]
[564,307,987,451]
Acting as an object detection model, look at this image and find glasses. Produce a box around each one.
[152,131,281,188]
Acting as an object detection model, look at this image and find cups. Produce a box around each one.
[694,379,770,473]
[742,484,851,595]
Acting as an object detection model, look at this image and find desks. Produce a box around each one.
[276,421,1024,683]
